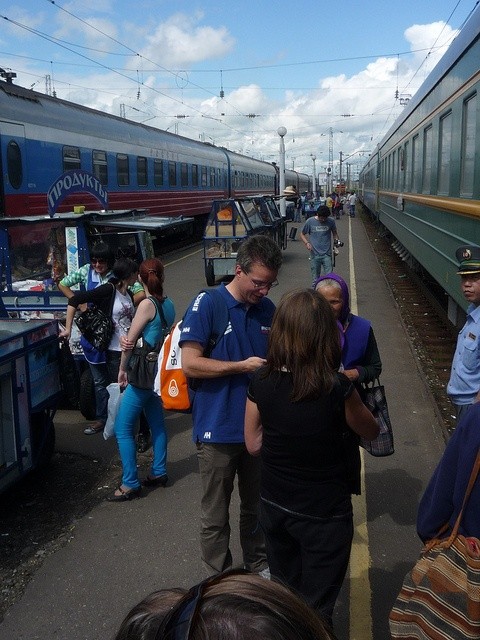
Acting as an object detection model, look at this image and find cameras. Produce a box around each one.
[334,238,344,249]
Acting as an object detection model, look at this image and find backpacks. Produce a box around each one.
[153,287,228,413]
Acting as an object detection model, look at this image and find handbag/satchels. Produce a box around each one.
[389,532,480,640]
[74,304,116,351]
[358,370,393,457]
[128,345,161,391]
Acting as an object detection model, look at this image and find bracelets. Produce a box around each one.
[305,241,309,245]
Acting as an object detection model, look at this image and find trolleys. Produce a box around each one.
[202,198,280,285]
[240,194,294,251]
[0,170,195,421]
[0,317,62,493]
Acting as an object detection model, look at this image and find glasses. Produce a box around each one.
[158,560,251,638]
[90,258,108,266]
[242,270,279,288]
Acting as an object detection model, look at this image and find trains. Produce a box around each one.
[0,68,332,275]
[354,1,480,330]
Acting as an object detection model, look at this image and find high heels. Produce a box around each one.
[106,482,143,502]
[141,473,168,488]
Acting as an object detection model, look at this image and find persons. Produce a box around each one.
[416,400,480,547]
[345,192,351,207]
[106,258,176,502]
[114,567,334,640]
[349,192,357,218]
[314,273,382,384]
[326,194,335,216]
[446,245,480,424]
[334,194,341,219]
[300,205,340,290]
[58,242,146,435]
[294,194,302,223]
[57,257,150,453]
[352,190,357,197]
[178,235,283,581]
[244,289,380,616]
[301,193,306,202]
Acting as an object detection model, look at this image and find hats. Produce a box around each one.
[456,245,480,276]
[281,185,295,197]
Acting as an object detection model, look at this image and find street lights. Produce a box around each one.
[346,162,350,189]
[324,165,327,199]
[277,127,288,222]
[340,149,372,194]
[312,155,317,198]
